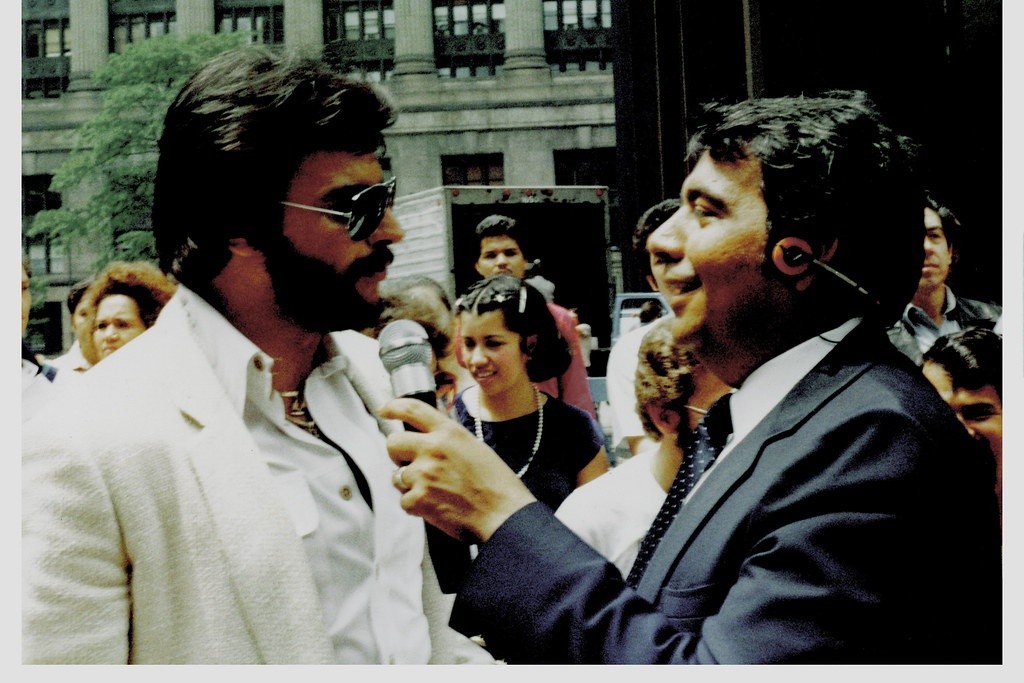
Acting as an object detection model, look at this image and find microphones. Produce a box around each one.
[378,320,474,595]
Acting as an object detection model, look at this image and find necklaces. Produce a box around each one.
[474,384,544,479]
[280,391,321,438]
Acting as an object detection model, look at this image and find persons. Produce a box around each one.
[606,199,682,459]
[886,188,1002,368]
[360,276,458,409]
[921,329,1002,501]
[553,323,733,582]
[448,275,610,518]
[22,44,492,664]
[473,215,598,419]
[22,247,56,382]
[379,91,1002,665]
[56,277,96,370]
[76,260,176,365]
[631,301,663,330]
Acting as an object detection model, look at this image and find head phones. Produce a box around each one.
[764,227,904,322]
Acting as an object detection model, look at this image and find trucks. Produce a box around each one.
[383,186,614,368]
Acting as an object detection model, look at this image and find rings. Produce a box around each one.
[396,466,407,488]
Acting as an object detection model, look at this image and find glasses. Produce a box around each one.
[272,176,397,242]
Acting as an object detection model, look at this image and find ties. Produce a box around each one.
[624,393,733,593]
